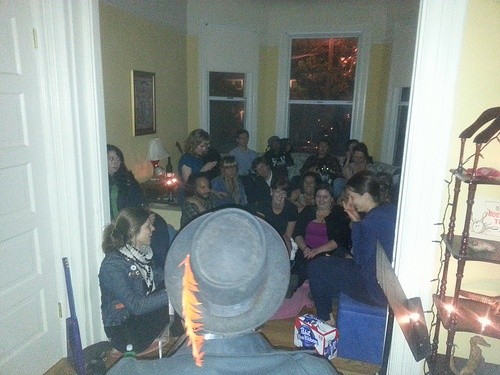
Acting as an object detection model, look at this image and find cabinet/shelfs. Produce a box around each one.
[421,107,500,375]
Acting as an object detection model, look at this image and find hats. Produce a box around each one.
[164,207,291,335]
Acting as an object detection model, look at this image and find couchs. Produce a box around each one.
[284,153,402,185]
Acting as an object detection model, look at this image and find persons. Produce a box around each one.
[101,208,339,375]
[98,129,397,354]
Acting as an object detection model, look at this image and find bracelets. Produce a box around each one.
[302,246,308,250]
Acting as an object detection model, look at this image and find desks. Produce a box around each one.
[139,203,184,231]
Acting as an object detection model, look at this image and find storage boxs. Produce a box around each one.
[292,313,338,362]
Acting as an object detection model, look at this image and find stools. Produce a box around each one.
[335,291,389,366]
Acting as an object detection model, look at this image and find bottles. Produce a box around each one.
[124,345,135,358]
[166,157,172,178]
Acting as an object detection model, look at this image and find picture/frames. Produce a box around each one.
[130,69,156,137]
